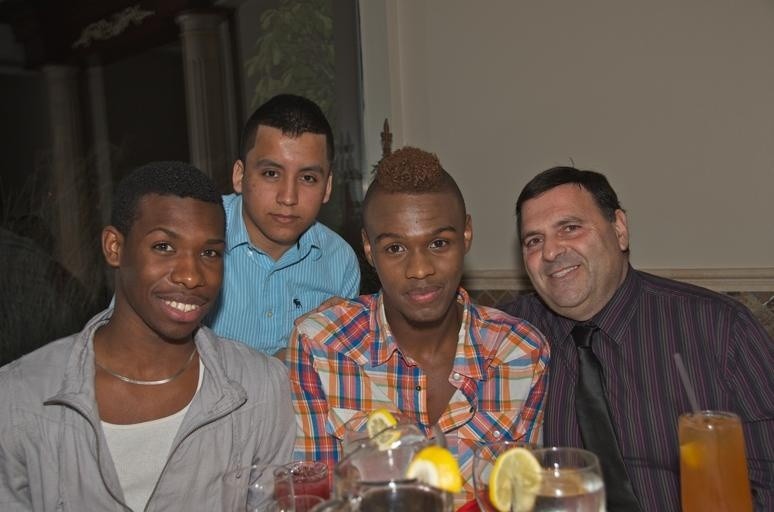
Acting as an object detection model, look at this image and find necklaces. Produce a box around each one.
[87,343,198,390]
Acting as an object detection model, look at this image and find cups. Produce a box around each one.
[270,494,324,511]
[673,410,752,512]
[272,459,333,512]
[514,449,606,511]
[313,410,455,511]
[471,441,529,511]
[223,463,297,511]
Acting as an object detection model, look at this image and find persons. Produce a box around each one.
[196,92,364,367]
[486,163,773,511]
[0,154,296,511]
[280,146,556,511]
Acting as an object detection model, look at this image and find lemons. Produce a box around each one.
[489,447,543,512]
[405,445,462,496]
[367,408,401,452]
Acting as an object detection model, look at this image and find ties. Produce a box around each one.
[570,323,642,512]
